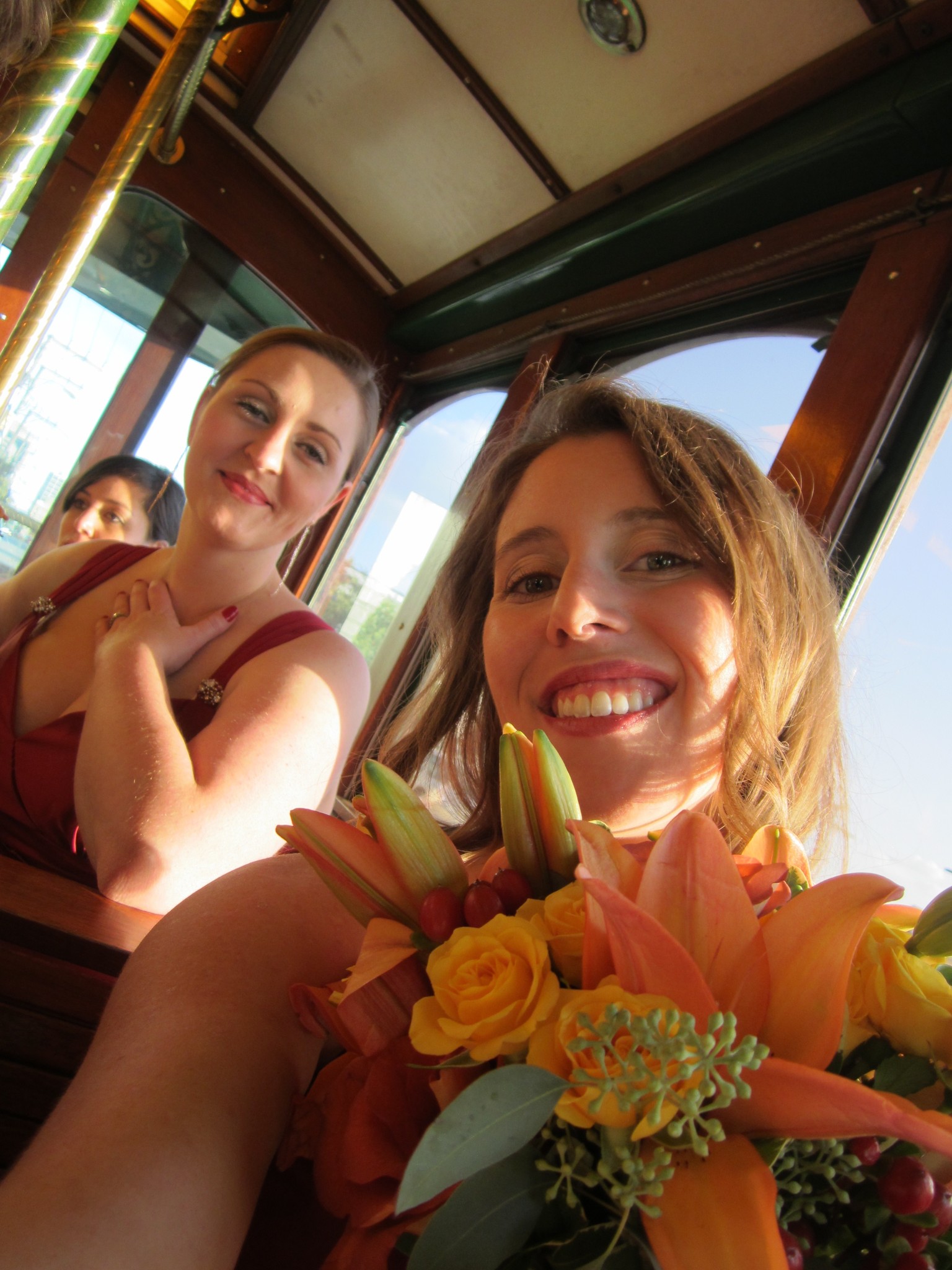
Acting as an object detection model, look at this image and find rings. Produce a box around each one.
[110,613,128,626]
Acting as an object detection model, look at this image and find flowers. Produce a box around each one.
[272,714,952,1270]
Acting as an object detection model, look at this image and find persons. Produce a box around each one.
[0,455,186,547]
[0,365,951,1270]
[0,326,379,917]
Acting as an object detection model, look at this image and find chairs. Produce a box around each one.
[0,850,168,1189]
